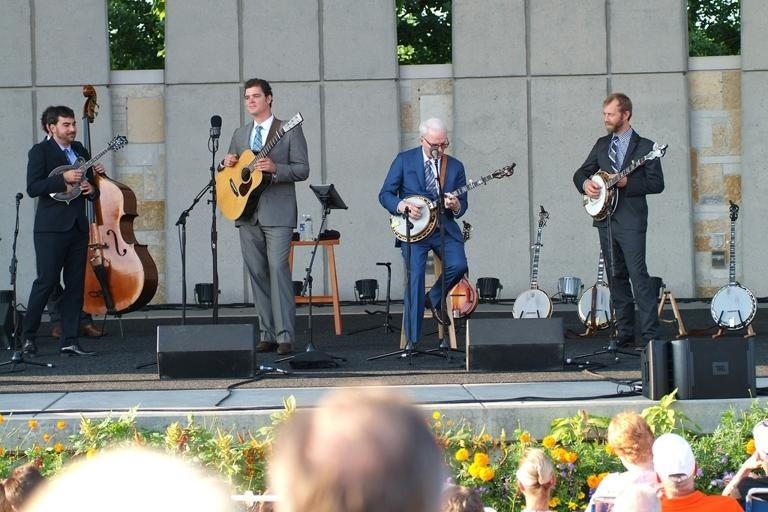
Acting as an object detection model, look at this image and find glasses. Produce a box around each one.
[420,133,451,150]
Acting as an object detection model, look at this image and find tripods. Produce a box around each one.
[403,165,465,362]
[0,205,55,373]
[274,212,347,367]
[565,211,641,365]
[367,211,454,365]
[350,265,401,336]
[132,185,212,370]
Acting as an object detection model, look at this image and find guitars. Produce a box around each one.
[206,111,309,226]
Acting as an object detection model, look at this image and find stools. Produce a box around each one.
[290,239,343,336]
[397,242,457,353]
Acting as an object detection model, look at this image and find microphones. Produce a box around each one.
[407,220,413,228]
[211,114,221,139]
[15,191,23,202]
[605,196,612,208]
[430,148,437,159]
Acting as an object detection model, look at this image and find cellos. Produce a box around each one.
[82,75,158,317]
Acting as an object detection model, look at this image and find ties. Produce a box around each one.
[608,135,618,168]
[63,149,72,166]
[424,160,437,199]
[252,124,262,153]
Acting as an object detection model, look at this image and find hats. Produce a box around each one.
[652,432,695,481]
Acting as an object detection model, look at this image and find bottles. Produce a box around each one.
[300,215,306,240]
[305,215,313,242]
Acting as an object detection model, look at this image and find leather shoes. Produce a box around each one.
[61,342,98,358]
[52,325,64,338]
[424,292,451,326]
[276,340,293,355]
[603,335,635,351]
[79,321,107,338]
[256,340,274,352]
[23,339,38,358]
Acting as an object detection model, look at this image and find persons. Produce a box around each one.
[650,433,743,511]
[445,485,482,512]
[21,106,106,359]
[573,93,666,358]
[2,464,47,511]
[379,118,469,359]
[720,418,768,511]
[584,411,663,512]
[218,79,310,355]
[42,106,103,339]
[514,445,560,512]
[265,382,443,512]
